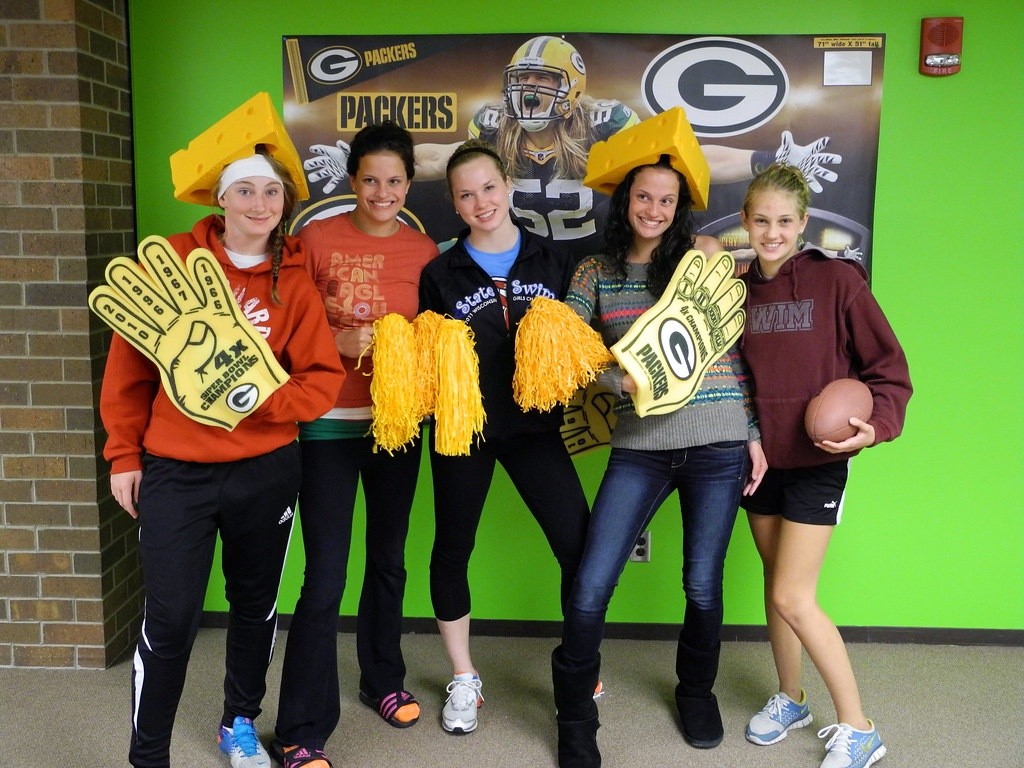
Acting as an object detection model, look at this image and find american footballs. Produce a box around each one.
[805,378,874,445]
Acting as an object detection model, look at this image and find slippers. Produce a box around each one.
[359,687,420,729]
[270,738,334,768]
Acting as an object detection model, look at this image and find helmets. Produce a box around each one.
[502,36,587,120]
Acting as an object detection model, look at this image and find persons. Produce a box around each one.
[303,35,843,268]
[98,119,915,767]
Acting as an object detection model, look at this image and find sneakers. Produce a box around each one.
[817,718,887,768]
[217,716,271,768]
[441,671,485,734]
[746,689,813,746]
[592,681,605,701]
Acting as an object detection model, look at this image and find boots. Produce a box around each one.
[552,644,601,768]
[673,633,724,749]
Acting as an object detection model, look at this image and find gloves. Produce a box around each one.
[837,245,864,263]
[86,234,292,434]
[609,249,748,418]
[304,140,352,195]
[751,131,843,194]
